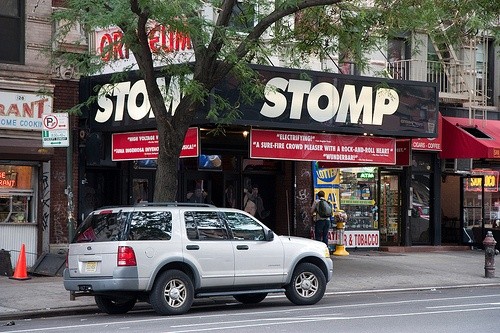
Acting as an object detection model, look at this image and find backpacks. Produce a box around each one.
[316,198,332,218]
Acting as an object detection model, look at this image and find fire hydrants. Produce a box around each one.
[482,231,499,278]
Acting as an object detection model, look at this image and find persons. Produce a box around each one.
[310,191,330,247]
[250,185,264,222]
[244,189,255,216]
[226,184,236,208]
[188,187,204,207]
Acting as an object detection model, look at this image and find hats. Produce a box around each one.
[195,183,201,189]
[317,191,325,197]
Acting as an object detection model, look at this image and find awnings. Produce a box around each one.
[439,116,500,158]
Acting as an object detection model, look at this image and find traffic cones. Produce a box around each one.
[8,244,32,281]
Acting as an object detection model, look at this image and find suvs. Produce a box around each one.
[62,200,334,317]
